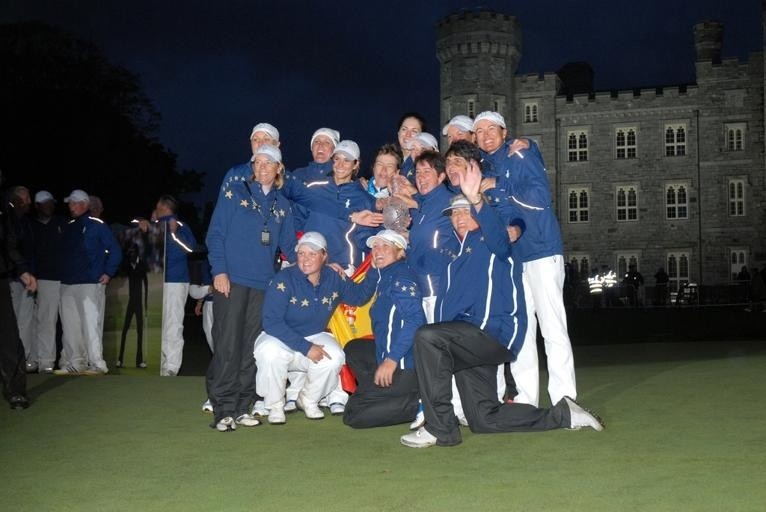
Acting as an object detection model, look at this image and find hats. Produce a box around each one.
[128,243,138,253]
[442,115,474,135]
[250,122,280,143]
[310,127,340,151]
[471,110,506,132]
[294,231,328,253]
[64,190,90,203]
[330,140,360,162]
[251,145,283,165]
[441,193,482,216]
[35,191,57,204]
[406,132,439,152]
[366,229,408,251]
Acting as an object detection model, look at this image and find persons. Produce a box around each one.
[737,266,751,303]
[138,196,215,377]
[1,184,121,411]
[203,111,604,448]
[114,243,149,367]
[750,267,766,303]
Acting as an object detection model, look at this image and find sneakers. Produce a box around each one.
[563,395,606,432]
[329,402,345,416]
[216,416,236,432]
[136,362,148,368]
[268,407,286,423]
[79,366,108,376]
[235,414,260,426]
[295,392,324,419]
[318,397,328,407]
[400,426,437,448]
[410,398,426,429]
[116,361,124,368]
[252,400,269,416]
[10,393,29,409]
[40,368,53,376]
[25,361,38,372]
[283,400,298,415]
[54,364,88,375]
[202,398,214,411]
